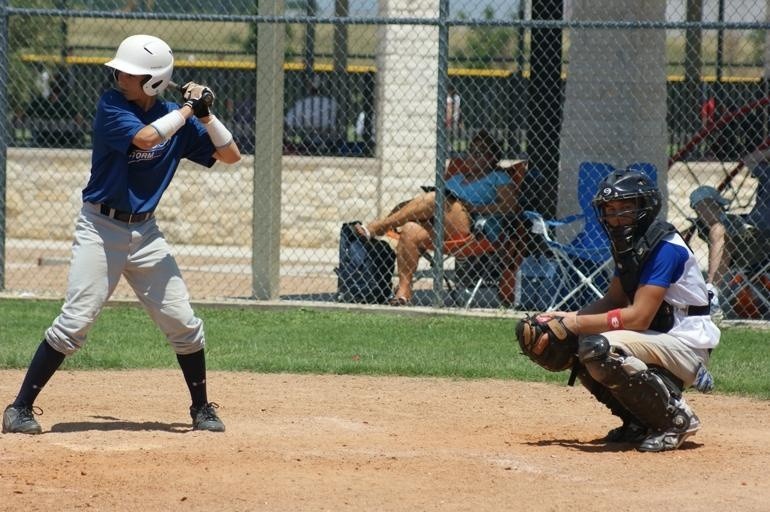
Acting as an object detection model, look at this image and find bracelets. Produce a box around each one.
[203,116,234,148]
[150,110,186,138]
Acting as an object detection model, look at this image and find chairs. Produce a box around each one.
[523,161,658,314]
[683,213,770,318]
[383,158,529,310]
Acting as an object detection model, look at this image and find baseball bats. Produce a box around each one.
[168,80,213,104]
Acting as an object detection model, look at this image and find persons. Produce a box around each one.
[2,34,243,433]
[351,130,520,305]
[286,72,339,130]
[516,169,722,453]
[11,71,87,145]
[689,182,770,317]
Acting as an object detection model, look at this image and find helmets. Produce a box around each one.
[591,167,664,256]
[105,33,173,97]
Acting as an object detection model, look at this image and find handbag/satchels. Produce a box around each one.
[336,220,396,303]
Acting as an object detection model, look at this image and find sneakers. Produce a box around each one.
[607,418,656,443]
[186,402,224,431]
[635,396,703,452]
[2,404,42,434]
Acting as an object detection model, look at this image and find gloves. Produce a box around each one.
[532,317,576,370]
[518,313,550,354]
[184,86,214,109]
[180,81,211,119]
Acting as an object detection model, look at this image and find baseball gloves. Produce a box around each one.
[517,312,577,371]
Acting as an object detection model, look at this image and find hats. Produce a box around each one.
[688,186,729,208]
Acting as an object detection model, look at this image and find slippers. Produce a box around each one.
[353,221,372,241]
[388,294,412,306]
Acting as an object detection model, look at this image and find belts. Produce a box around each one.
[680,304,711,315]
[97,203,159,224]
